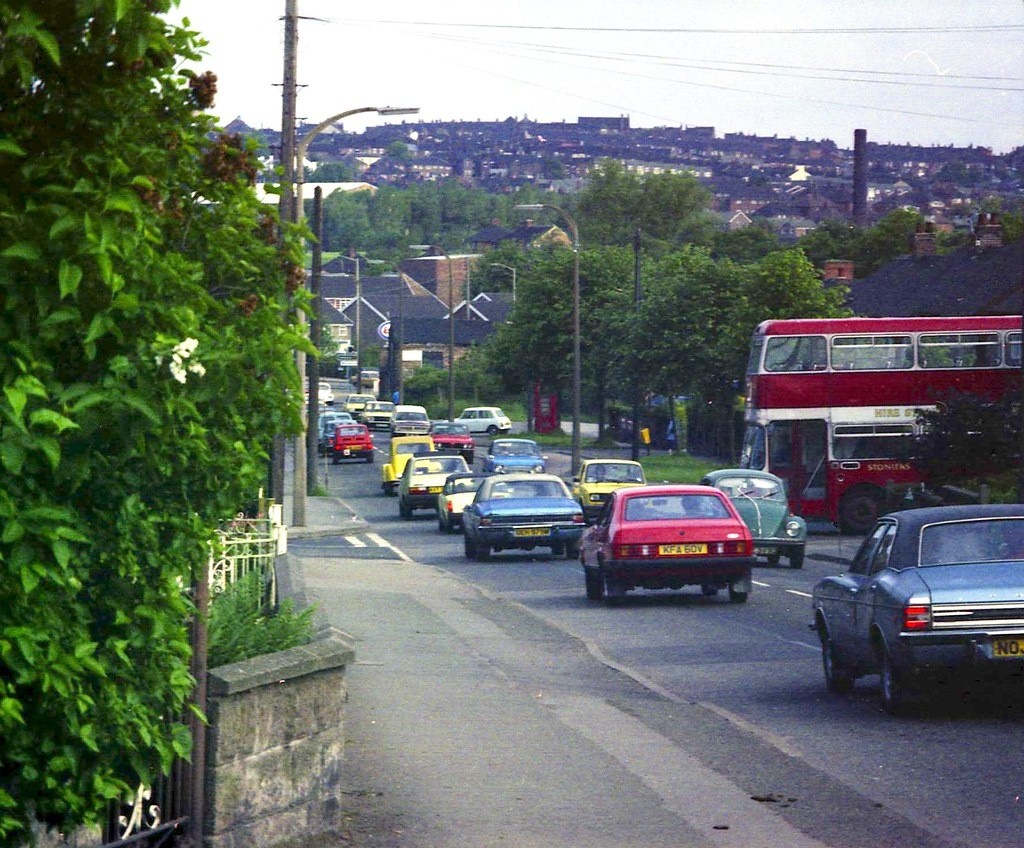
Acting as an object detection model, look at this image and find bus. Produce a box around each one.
[738,316,1024,539]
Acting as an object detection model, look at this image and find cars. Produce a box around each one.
[807,503,1024,719]
[438,406,512,436]
[701,468,810,568]
[571,483,757,605]
[460,471,588,562]
[439,470,513,535]
[395,450,474,519]
[382,434,442,498]
[310,369,434,464]
[479,438,549,481]
[426,419,476,464]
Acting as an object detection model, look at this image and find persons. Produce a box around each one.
[664,413,675,456]
[971,345,990,367]
[777,432,793,460]
[592,465,608,483]
[639,423,651,456]
[392,386,400,405]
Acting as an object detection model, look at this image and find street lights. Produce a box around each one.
[514,202,583,477]
[292,105,421,527]
[409,244,455,423]
[336,254,360,395]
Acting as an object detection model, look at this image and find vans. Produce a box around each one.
[570,458,647,529]
